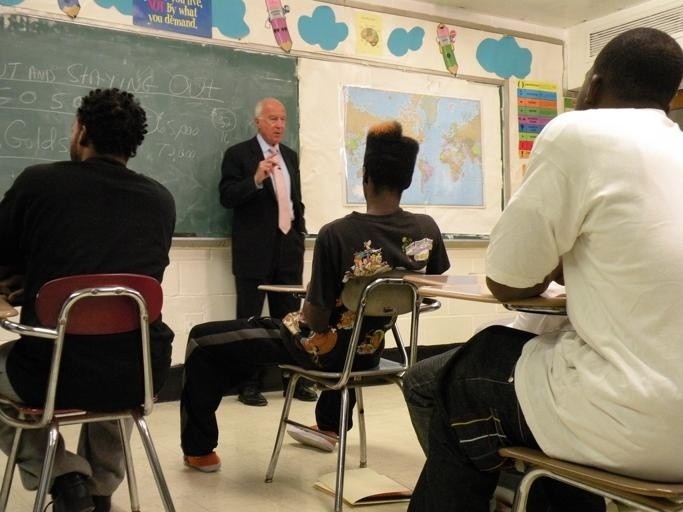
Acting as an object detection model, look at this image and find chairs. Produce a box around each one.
[499,447,683,512]
[265,273,441,512]
[0,273,175,512]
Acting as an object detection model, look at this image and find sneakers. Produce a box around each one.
[183,451,221,472]
[286,424,337,452]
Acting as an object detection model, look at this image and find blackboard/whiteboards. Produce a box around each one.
[0,6,507,247]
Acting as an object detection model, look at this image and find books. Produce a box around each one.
[311,466,412,506]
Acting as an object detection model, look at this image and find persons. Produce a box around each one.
[0,82,179,510]
[176,118,452,473]
[214,93,318,407]
[403,26,683,510]
[398,269,584,512]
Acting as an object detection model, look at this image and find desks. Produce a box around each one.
[404,282,568,310]
[258,283,318,295]
[402,274,557,285]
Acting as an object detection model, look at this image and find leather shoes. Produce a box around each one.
[48,471,111,512]
[238,387,268,406]
[283,381,318,401]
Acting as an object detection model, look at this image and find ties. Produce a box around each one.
[268,148,291,235]
